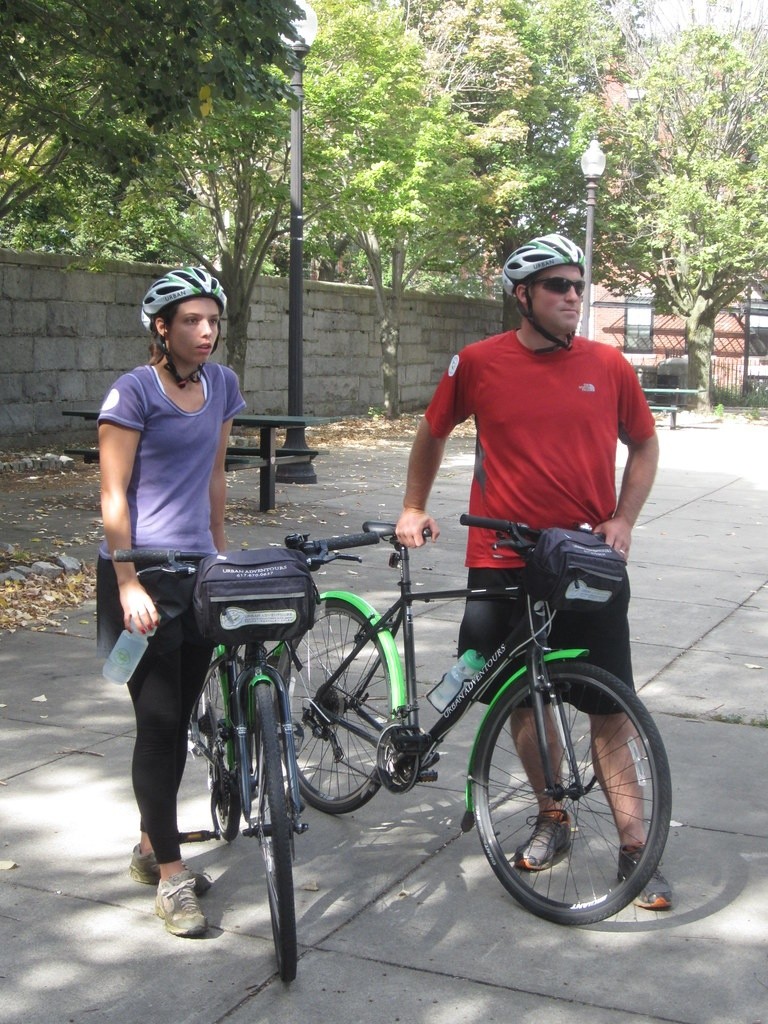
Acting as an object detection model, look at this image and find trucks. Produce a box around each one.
[729,298,768,392]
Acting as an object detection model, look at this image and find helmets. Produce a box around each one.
[501,234,586,296]
[140,267,227,336]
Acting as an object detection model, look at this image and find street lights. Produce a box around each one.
[578,139,606,338]
[277,0,320,485]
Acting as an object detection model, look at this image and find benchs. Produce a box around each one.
[650,402,690,411]
[225,447,322,458]
[65,445,261,473]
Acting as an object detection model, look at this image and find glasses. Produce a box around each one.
[529,277,586,296]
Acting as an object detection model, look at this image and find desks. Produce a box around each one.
[60,410,343,512]
[642,388,706,429]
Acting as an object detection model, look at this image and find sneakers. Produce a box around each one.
[155,870,208,936]
[617,846,672,909]
[129,843,212,895]
[514,809,571,870]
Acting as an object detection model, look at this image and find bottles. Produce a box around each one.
[101,603,162,685]
[431,649,486,708]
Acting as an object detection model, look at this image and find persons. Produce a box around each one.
[97,268,247,939]
[394,234,673,909]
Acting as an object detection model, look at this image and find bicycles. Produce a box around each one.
[273,514,674,927]
[110,528,380,985]
